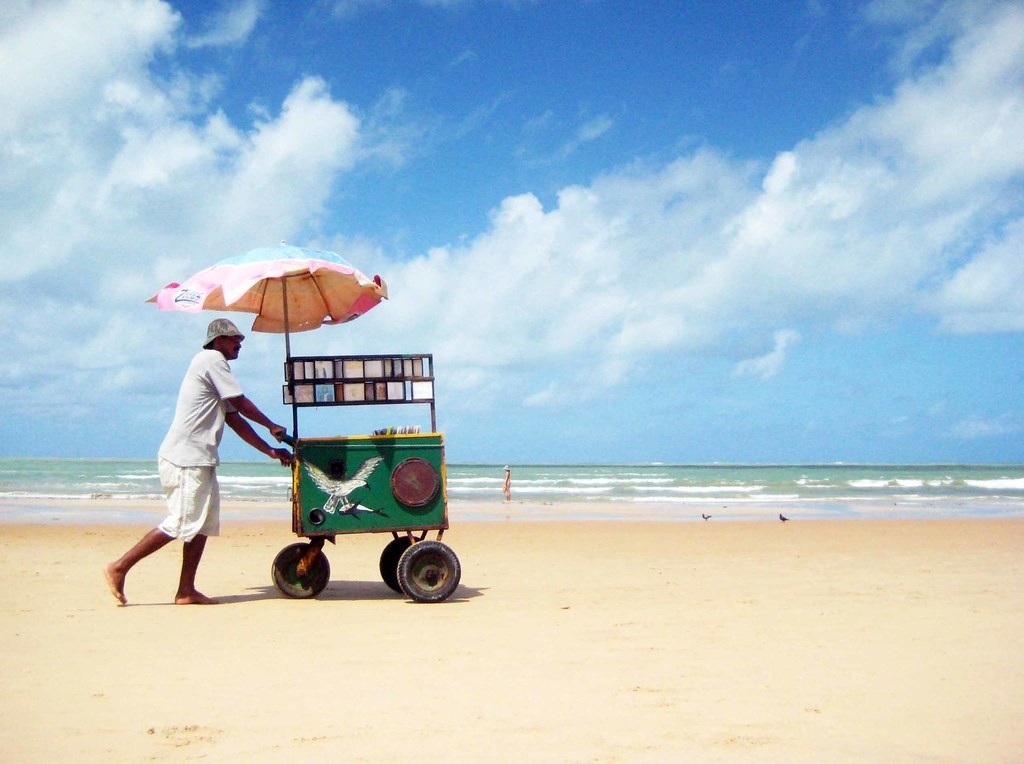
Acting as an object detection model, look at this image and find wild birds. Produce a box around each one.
[779,513,789,522]
[702,513,712,520]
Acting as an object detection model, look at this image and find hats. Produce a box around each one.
[203,319,245,349]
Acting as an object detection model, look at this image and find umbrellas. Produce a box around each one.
[147,242,388,390]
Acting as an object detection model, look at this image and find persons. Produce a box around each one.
[105,320,290,606]
[503,465,511,503]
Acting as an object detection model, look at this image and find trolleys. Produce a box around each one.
[270,353,461,603]
